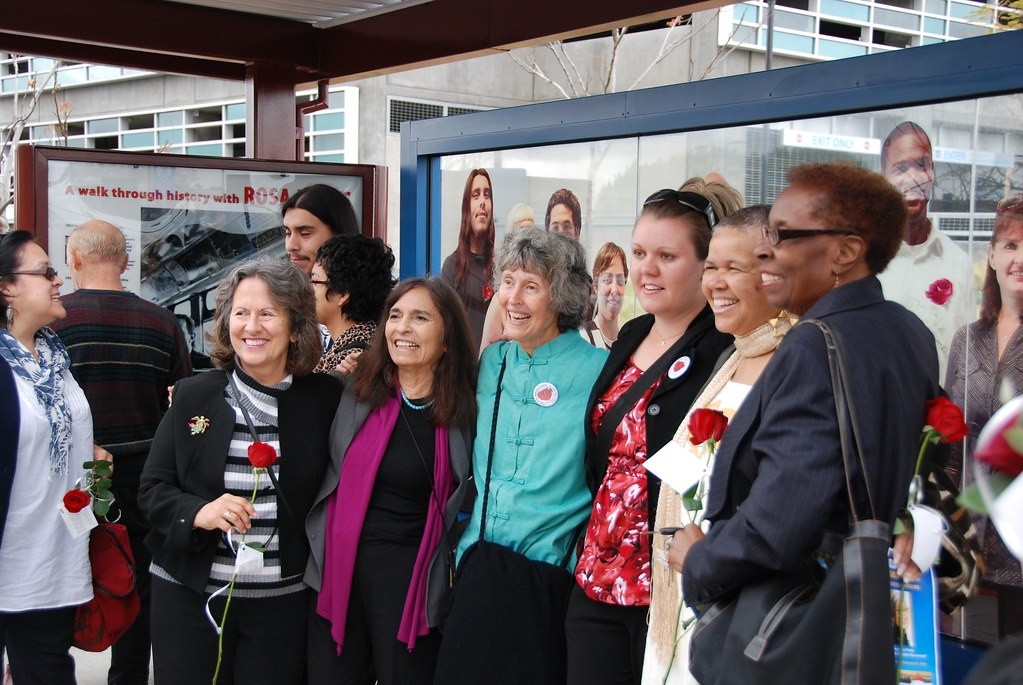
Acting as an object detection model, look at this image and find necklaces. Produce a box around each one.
[399,387,436,409]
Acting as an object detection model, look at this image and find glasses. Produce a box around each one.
[761,225,855,247]
[7,267,59,282]
[642,189,715,229]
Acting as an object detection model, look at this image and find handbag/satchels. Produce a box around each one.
[689,520,900,684]
[73,523,142,652]
[432,541,572,685]
[428,542,457,629]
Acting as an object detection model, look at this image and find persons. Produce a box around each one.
[309,234,398,375]
[639,203,922,685]
[336,229,611,685]
[579,243,628,357]
[473,203,534,360]
[942,193,1023,533]
[440,168,496,360]
[542,187,583,249]
[0,229,113,684]
[303,277,483,685]
[557,187,735,685]
[664,163,942,685]
[55,220,194,684]
[136,254,344,685]
[876,121,977,392]
[281,184,359,280]
[170,312,217,369]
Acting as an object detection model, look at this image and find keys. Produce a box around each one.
[638,527,684,536]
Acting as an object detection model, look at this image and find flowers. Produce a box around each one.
[662,409,729,685]
[926,279,953,304]
[896,396,1023,685]
[63,460,113,515]
[483,285,494,303]
[213,441,278,685]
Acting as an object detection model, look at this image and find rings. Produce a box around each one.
[666,537,673,551]
[225,511,232,520]
